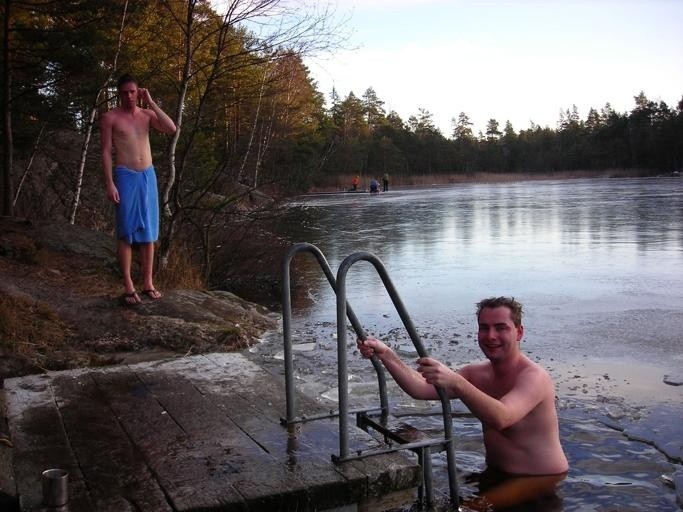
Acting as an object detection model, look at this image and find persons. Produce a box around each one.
[382,168,389,192]
[100,73,177,306]
[352,176,360,189]
[356,296,569,511]
[369,177,378,191]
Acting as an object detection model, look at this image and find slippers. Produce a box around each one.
[124,290,142,306]
[141,287,165,301]
